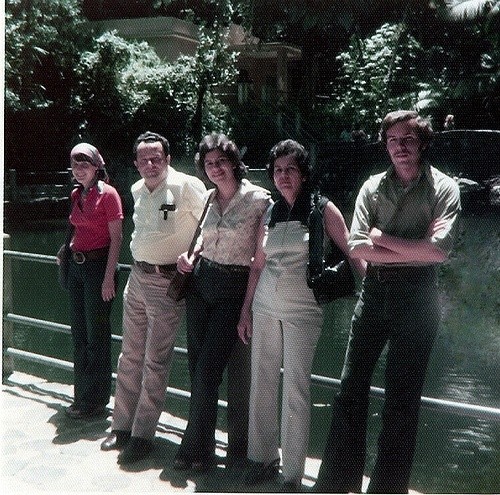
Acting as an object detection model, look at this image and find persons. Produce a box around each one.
[59,142,123,419]
[101,132,209,461]
[171,131,276,469]
[244,139,367,495]
[312,110,461,494]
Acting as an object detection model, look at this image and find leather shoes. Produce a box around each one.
[120,436,152,462]
[101,429,130,451]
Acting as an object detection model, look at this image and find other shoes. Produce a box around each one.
[65,403,108,419]
[173,455,212,471]
[279,482,300,493]
[244,458,279,486]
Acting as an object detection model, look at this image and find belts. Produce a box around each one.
[201,258,250,275]
[70,250,108,265]
[365,267,436,283]
[134,261,178,275]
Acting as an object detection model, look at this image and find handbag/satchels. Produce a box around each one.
[307,197,357,305]
[166,266,193,302]
[57,248,71,289]
